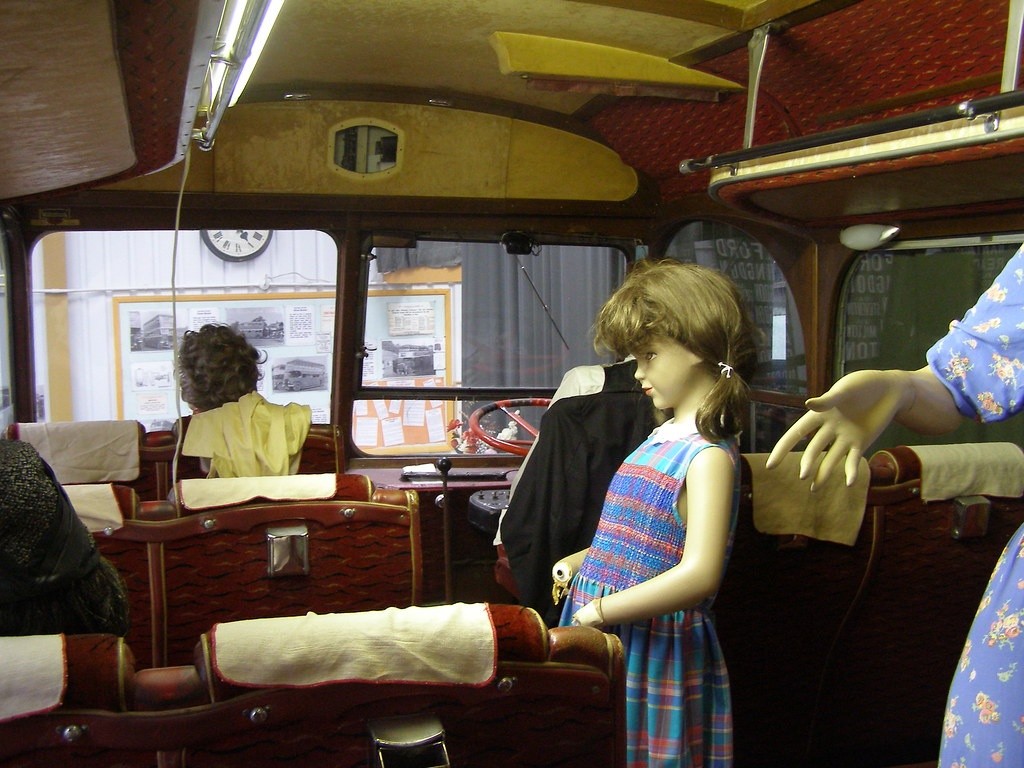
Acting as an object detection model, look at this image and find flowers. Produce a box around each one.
[448,411,500,456]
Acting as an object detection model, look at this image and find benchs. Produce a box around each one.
[0,361,1024,768]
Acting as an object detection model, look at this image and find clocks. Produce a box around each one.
[200,230,273,262]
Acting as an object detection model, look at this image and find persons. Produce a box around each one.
[557,259,758,768]
[0,439,128,635]
[762,236,1023,768]
[175,319,268,412]
